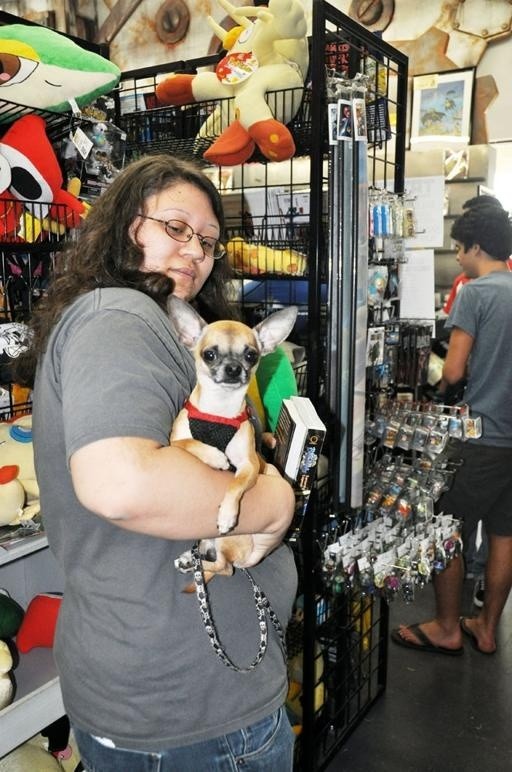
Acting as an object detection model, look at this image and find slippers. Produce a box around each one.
[394,621,462,657]
[460,611,482,652]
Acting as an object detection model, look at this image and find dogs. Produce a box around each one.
[165,292,300,594]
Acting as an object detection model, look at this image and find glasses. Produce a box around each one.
[138,212,226,260]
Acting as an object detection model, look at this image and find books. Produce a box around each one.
[267,395,328,547]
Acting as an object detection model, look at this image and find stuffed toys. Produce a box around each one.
[0,19,122,126]
[2,410,43,532]
[0,580,64,716]
[1,112,97,246]
[154,0,313,166]
[220,236,327,327]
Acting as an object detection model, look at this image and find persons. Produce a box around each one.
[438,191,512,612]
[328,103,367,137]
[388,201,512,659]
[30,152,303,771]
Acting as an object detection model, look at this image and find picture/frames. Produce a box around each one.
[411,65,476,147]
